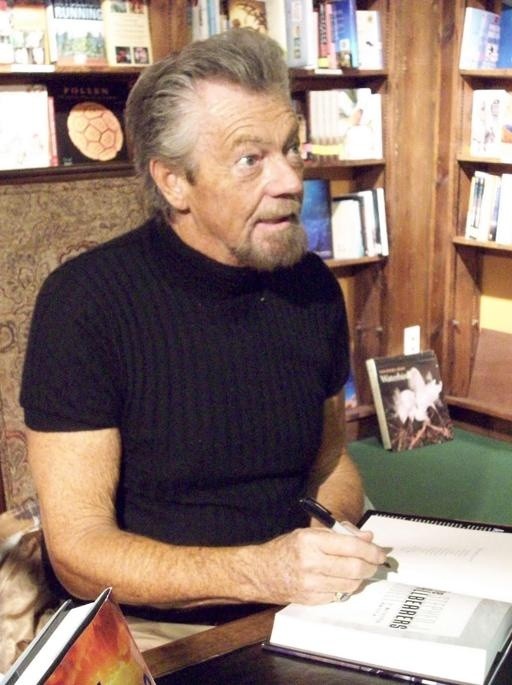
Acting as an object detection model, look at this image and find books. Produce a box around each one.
[363,350,453,451]
[2,587,157,685]
[191,0,390,258]
[1,0,153,170]
[262,510,510,685]
[459,7,512,249]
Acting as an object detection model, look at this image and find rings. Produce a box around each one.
[335,592,348,602]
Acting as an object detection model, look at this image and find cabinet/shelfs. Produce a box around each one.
[425,0,511,441]
[0,2,404,441]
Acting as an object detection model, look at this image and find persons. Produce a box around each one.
[16,26,391,645]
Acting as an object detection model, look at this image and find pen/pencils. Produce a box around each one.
[299,496,393,571]
